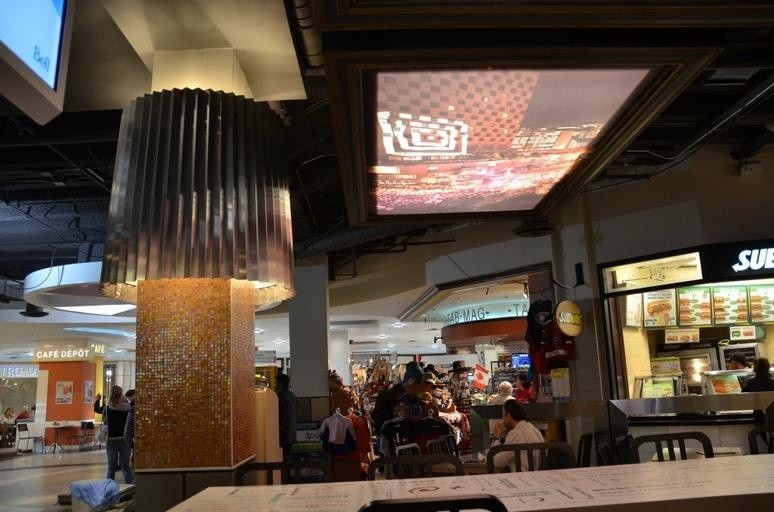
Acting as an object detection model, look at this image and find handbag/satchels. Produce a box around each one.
[98,425,108,443]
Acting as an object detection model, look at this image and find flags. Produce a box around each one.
[471,364,489,391]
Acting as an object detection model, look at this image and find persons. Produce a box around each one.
[15,405,30,425]
[488,399,550,472]
[486,379,516,438]
[0,408,17,424]
[514,373,536,405]
[730,350,751,370]
[273,374,297,484]
[94,386,135,484]
[742,358,774,392]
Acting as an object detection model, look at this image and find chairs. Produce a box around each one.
[232,460,332,484]
[358,493,507,511]
[14,424,43,455]
[628,430,714,462]
[74,422,103,452]
[366,452,465,479]
[382,428,607,476]
[745,420,773,452]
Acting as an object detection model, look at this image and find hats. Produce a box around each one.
[402,361,446,384]
[731,352,751,367]
[448,360,471,373]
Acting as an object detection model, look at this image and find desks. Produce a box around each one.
[160,448,774,512]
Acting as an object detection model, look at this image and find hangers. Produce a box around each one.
[536,290,547,302]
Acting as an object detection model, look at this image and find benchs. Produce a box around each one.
[68,481,137,512]
